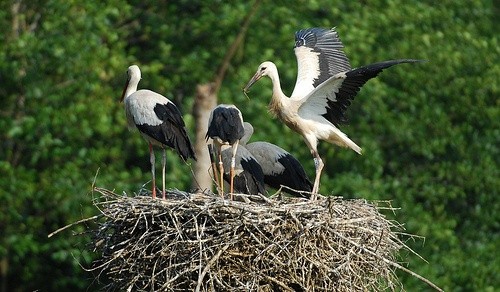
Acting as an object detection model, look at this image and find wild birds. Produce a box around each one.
[244,28,423,201]
[205,104,243,200]
[238,122,314,201]
[214,138,265,204]
[120,65,198,201]
